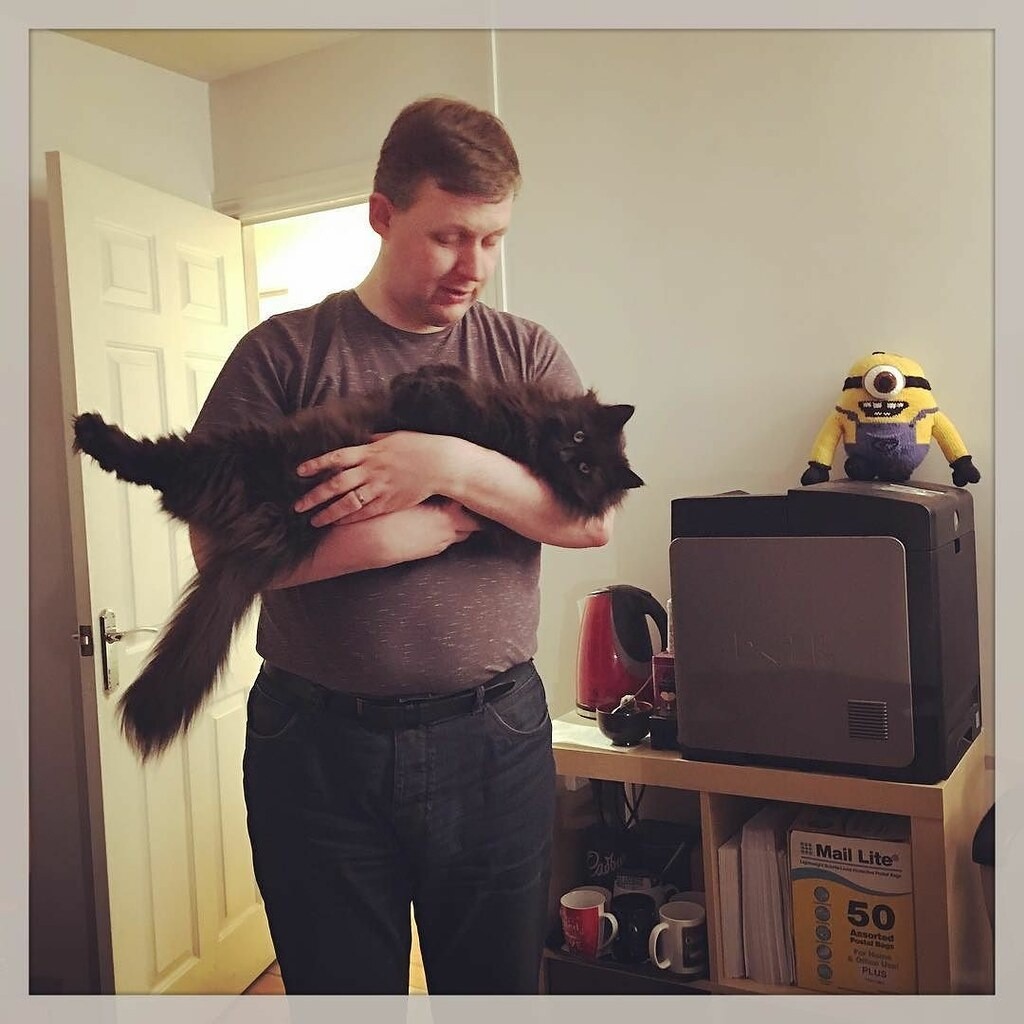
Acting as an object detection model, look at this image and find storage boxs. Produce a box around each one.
[787,806,919,997]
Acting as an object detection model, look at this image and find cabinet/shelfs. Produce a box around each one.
[552,706,995,998]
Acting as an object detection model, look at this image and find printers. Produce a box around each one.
[669,476,982,785]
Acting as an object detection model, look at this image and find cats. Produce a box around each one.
[70,362,644,761]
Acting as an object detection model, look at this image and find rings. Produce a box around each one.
[353,487,368,507]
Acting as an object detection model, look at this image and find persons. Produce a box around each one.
[186,98,626,994]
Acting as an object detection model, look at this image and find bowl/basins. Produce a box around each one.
[594,700,656,747]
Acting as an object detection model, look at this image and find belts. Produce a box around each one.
[261,658,536,725]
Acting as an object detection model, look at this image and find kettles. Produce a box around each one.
[573,583,669,717]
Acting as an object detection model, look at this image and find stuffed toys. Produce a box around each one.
[800,350,981,488]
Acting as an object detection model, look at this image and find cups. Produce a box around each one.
[558,876,709,974]
